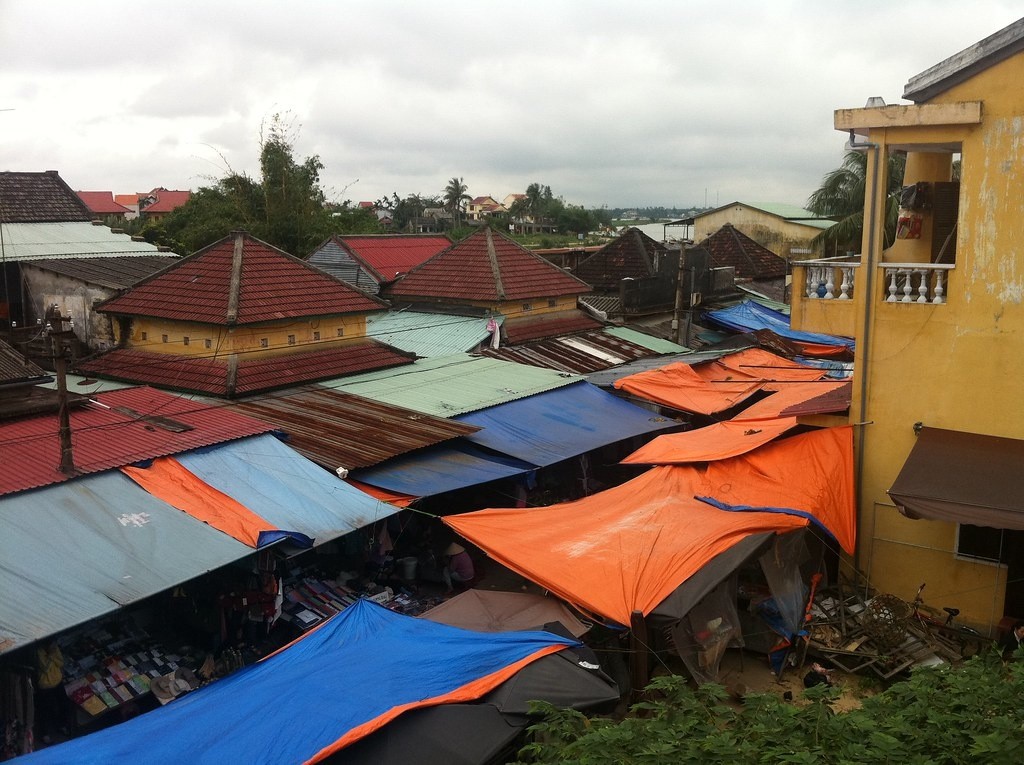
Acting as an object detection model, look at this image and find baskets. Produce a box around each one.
[862,594,912,646]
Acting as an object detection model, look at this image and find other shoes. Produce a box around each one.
[443,590,454,595]
[40,735,54,745]
[58,726,70,736]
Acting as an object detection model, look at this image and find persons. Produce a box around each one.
[443,543,475,594]
[1001,621,1024,655]
[36,636,69,744]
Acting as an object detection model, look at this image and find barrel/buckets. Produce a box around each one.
[396,557,418,579]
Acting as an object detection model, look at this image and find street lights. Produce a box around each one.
[669,238,694,349]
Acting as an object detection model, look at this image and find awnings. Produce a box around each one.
[1,301,870,765]
[890,426,1024,530]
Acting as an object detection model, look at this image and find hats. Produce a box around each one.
[175,667,196,688]
[151,677,173,698]
[169,679,191,696]
[444,542,465,555]
